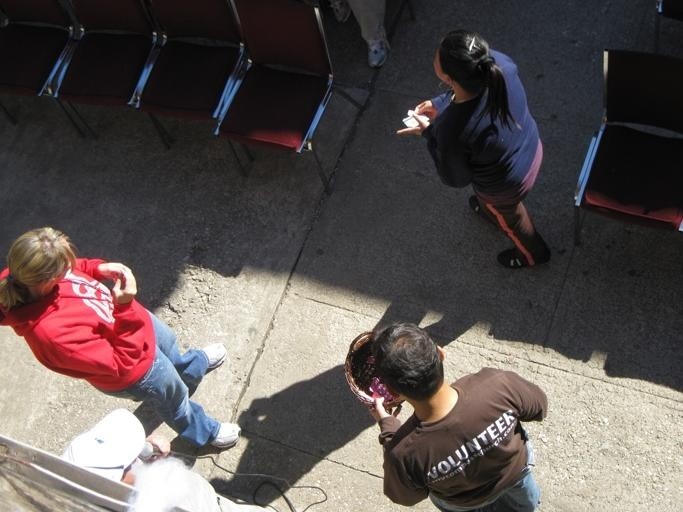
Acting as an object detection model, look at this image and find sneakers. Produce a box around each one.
[469,196,482,212]
[209,423,241,449]
[498,246,551,267]
[203,343,227,368]
[367,36,387,69]
[331,1,351,22]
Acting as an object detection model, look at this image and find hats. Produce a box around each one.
[62,408,146,482]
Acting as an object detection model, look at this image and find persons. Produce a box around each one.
[367,324,547,511]
[346,0,391,68]
[390,28,551,267]
[0,226,240,451]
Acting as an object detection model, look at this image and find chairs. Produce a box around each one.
[569,46,682,248]
